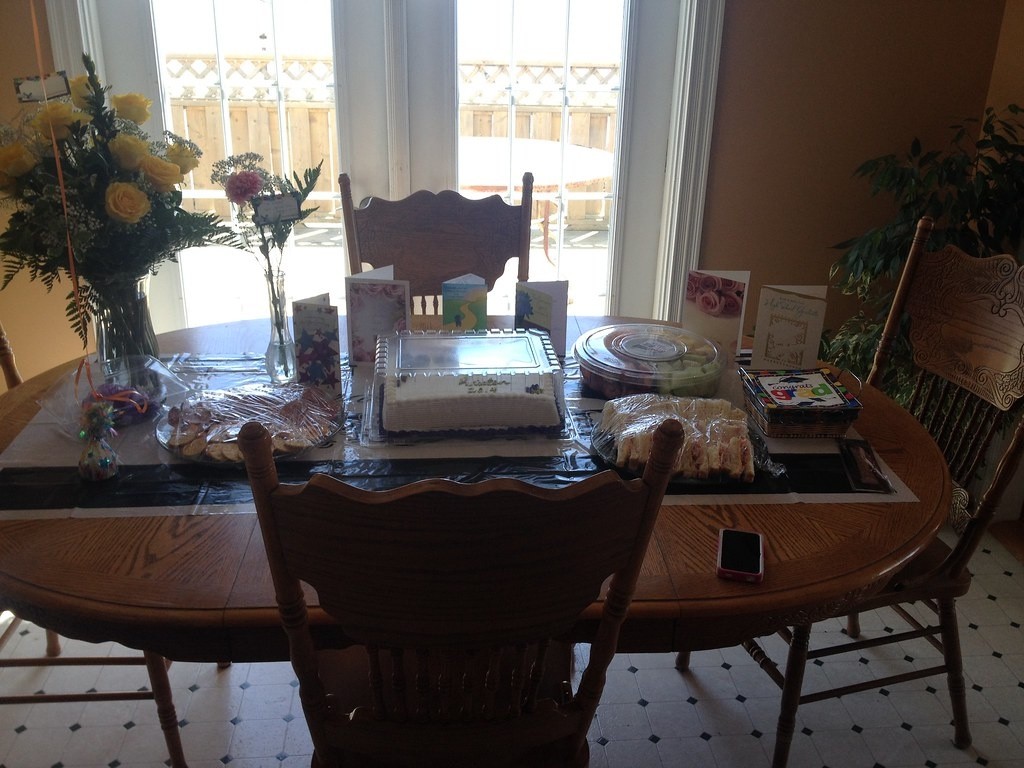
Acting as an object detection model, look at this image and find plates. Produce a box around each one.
[154,390,347,462]
[591,420,768,486]
[571,322,728,400]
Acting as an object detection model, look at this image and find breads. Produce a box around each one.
[599,393,755,483]
[168,384,337,463]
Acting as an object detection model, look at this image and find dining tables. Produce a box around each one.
[0,314,948,669]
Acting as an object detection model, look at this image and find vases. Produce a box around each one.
[87,291,167,409]
[262,268,301,383]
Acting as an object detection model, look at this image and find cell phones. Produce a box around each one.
[716,528,764,583]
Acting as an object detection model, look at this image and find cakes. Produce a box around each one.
[381,344,561,433]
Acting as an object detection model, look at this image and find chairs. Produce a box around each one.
[674,216,1024,768]
[238,419,684,767]
[337,172,533,316]
[0,326,187,768]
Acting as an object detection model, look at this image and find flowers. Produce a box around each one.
[0,54,253,395]
[209,147,325,376]
